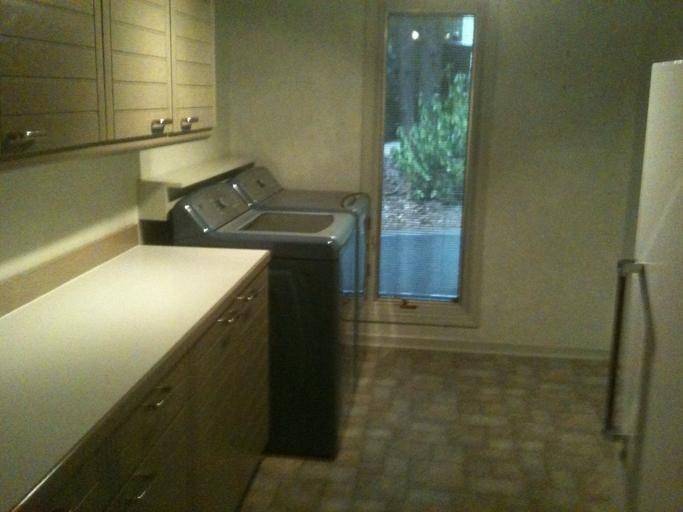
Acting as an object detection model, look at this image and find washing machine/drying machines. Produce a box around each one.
[167,181,359,460]
[229,165,371,387]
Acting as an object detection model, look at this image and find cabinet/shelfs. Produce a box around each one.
[0,265,269,512]
[0,0,107,171]
[102,1,218,154]
